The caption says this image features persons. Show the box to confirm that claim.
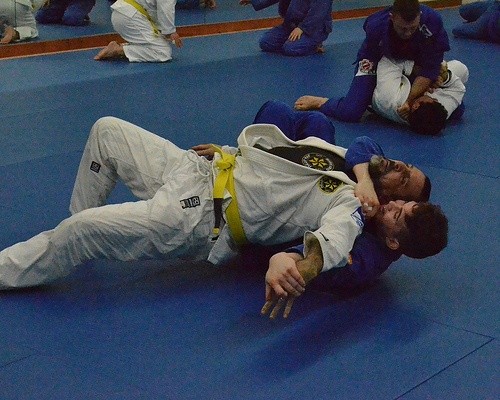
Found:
[94,0,183,62]
[294,0,451,122]
[372,54,469,136]
[452,0,500,42]
[36,0,95,26]
[253,100,449,301]
[0,116,431,318]
[240,0,333,56]
[0,0,39,44]
